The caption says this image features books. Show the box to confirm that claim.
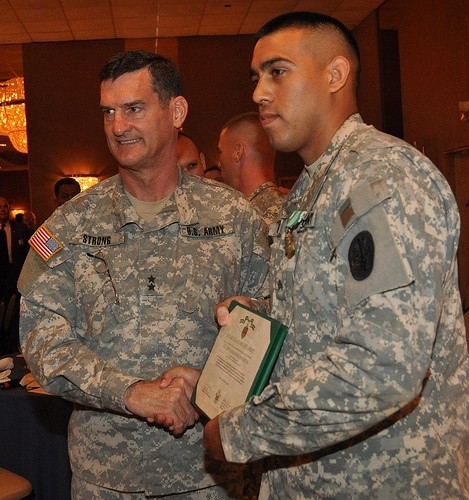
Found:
[190,299,288,424]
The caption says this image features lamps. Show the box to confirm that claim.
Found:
[65,176,98,193]
[0,77,28,153]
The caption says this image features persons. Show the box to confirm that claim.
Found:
[54,178,80,207]
[0,197,36,356]
[203,11,469,500]
[17,49,272,500]
[217,112,288,224]
[177,132,205,178]
